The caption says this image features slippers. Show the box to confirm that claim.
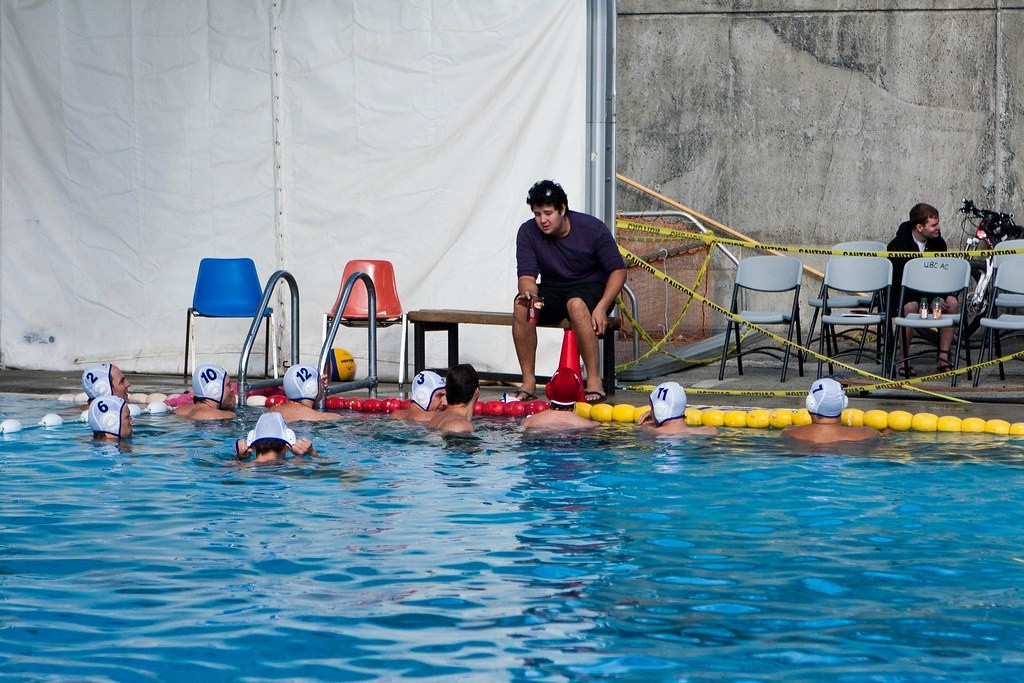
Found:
[513,390,539,400]
[898,366,917,376]
[585,390,607,403]
[937,365,952,373]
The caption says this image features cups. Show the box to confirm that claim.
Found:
[528,296,543,324]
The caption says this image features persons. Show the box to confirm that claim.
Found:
[174,364,237,419]
[389,371,448,421]
[887,203,959,376]
[81,363,130,402]
[269,363,342,423]
[429,363,480,432]
[88,395,134,439]
[235,411,316,461]
[780,377,882,443]
[521,367,600,428]
[508,180,625,402]
[637,382,719,436]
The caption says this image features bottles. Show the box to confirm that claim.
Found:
[920,298,929,319]
[933,297,941,319]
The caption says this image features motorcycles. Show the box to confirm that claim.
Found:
[914,199,1024,345]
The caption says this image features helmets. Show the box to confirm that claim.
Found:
[330,348,355,383]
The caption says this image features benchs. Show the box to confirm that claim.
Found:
[408,307,622,397]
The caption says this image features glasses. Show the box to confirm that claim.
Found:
[528,189,552,199]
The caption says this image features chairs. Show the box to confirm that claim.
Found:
[322,258,408,386]
[185,257,279,376]
[817,256,895,380]
[889,258,976,387]
[973,260,1024,388]
[718,255,805,382]
[988,238,1024,360]
[802,242,887,364]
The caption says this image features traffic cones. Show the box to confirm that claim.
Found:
[559,329,585,403]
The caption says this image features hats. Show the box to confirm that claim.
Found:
[283,364,319,401]
[89,395,126,435]
[82,363,113,401]
[650,382,687,425]
[545,367,583,405]
[411,371,446,411]
[192,364,227,403]
[806,378,849,416]
[246,412,296,449]
